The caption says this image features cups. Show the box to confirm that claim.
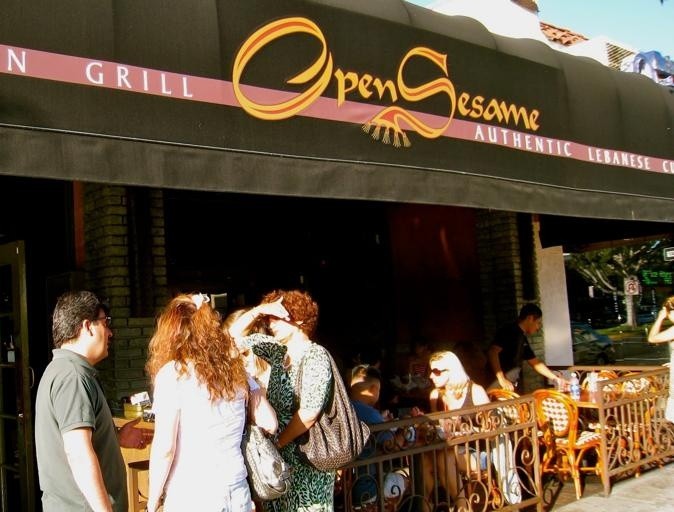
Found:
[130,390,152,422]
[587,372,598,391]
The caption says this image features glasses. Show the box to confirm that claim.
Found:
[88,315,112,327]
[427,368,446,376]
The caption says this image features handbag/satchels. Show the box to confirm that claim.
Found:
[292,344,371,472]
[241,424,292,502]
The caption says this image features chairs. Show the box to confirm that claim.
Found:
[486,370,659,501]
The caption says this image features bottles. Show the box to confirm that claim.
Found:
[554,373,565,393]
[569,373,581,402]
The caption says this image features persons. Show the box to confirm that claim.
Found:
[641,290,674,432]
[220,305,275,392]
[145,291,258,512]
[230,287,342,512]
[487,302,573,397]
[29,287,133,512]
[345,363,413,506]
[411,349,516,512]
